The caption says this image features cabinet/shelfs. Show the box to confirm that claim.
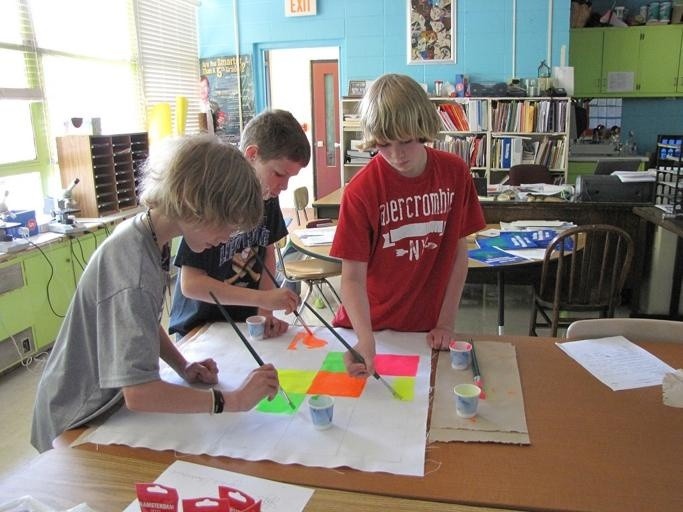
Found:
[339,96,570,185]
[56,132,150,218]
[568,155,650,185]
[568,24,683,98]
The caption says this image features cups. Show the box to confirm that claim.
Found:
[307,395,335,432]
[453,382,482,419]
[434,80,443,97]
[448,341,473,371]
[245,316,267,342]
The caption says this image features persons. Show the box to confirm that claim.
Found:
[328,72,487,379]
[168,108,311,342]
[29,129,280,453]
[200,75,220,133]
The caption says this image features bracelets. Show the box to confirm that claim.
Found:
[210,387,225,415]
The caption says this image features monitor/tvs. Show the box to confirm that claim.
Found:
[593,158,641,175]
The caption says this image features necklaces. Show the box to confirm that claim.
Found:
[146,207,171,317]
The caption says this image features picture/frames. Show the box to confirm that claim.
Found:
[406,0,457,65]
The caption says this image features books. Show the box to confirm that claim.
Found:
[343,100,567,170]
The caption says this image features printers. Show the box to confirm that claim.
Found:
[576,175,657,202]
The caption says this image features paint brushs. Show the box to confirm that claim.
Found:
[304,301,400,399]
[470,338,486,400]
[254,250,314,336]
[210,292,295,410]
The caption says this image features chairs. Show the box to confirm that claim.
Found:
[566,318,683,341]
[275,235,342,326]
[294,186,309,225]
[529,225,635,338]
[509,164,550,185]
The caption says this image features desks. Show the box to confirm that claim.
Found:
[0,449,519,511]
[312,187,652,284]
[632,207,683,316]
[284,222,586,328]
[50,321,683,511]
